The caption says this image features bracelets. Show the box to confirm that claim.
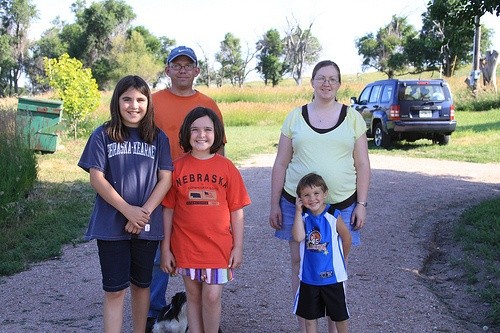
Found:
[356,201,368,207]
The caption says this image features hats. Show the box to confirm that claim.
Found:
[166,45,197,65]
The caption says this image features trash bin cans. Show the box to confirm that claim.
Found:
[16,96,61,153]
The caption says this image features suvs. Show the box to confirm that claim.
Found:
[349,78,457,149]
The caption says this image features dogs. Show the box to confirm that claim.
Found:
[154,291,189,333]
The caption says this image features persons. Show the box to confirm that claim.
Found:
[160,106,252,333]
[290,173,352,333]
[270,60,370,333]
[78,75,175,333]
[145,46,227,333]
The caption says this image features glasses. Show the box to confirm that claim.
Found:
[315,76,338,85]
[169,64,197,72]
